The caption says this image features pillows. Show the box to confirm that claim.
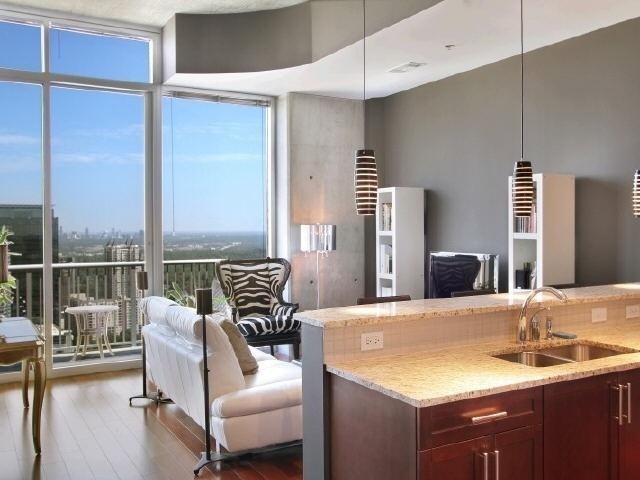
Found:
[214,315,259,376]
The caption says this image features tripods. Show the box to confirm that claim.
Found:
[128,291,161,405]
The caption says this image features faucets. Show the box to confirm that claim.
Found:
[514,286,569,344]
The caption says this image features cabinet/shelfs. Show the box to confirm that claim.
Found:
[375,185,425,300]
[507,173,576,292]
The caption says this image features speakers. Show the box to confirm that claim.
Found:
[137,271,149,289]
[196,289,213,314]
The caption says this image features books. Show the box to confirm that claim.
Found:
[514,206,536,290]
[380,202,396,296]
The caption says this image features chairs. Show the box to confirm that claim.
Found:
[429,252,481,298]
[216,258,301,360]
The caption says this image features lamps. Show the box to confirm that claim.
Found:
[631,170,640,219]
[300,223,337,311]
[129,272,159,408]
[511,1,533,217]
[353,0,378,216]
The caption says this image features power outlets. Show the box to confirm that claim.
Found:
[360,331,384,351]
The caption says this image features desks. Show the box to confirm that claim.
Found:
[1,316,46,453]
[64,304,120,362]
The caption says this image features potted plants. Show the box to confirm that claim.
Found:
[0,224,17,308]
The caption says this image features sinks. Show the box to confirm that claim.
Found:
[487,341,573,368]
[534,334,640,364]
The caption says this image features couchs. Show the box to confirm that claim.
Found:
[138,296,302,456]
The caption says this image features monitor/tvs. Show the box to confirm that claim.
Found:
[427,251,499,300]
